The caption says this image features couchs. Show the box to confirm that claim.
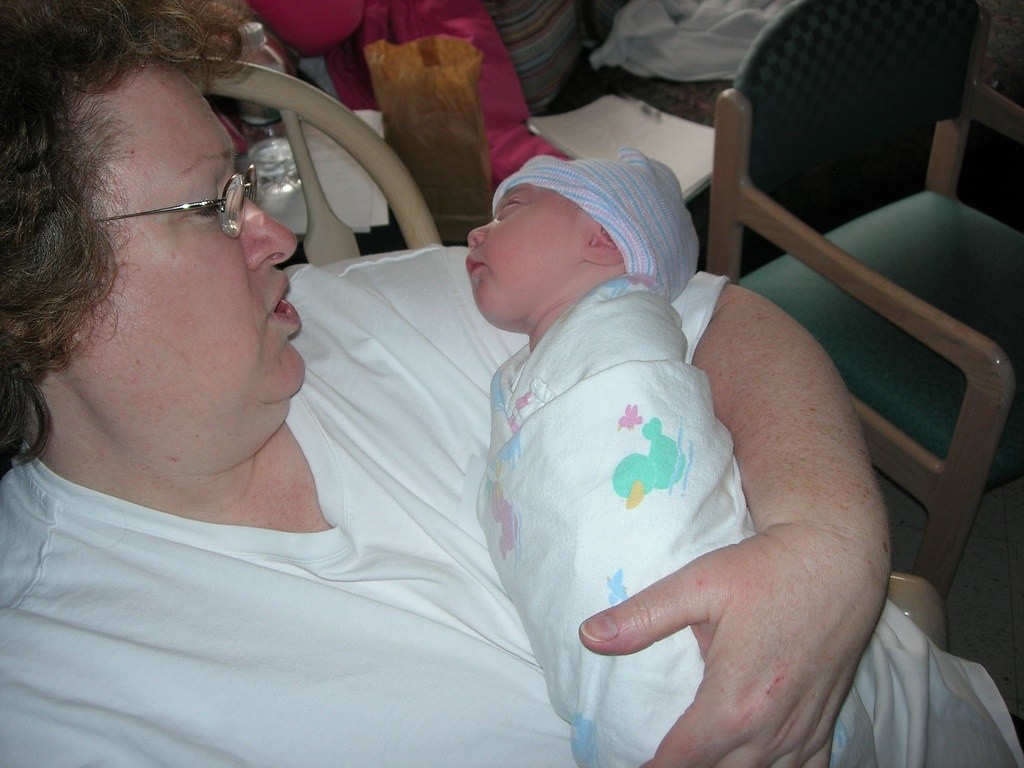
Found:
[481,0,1024,274]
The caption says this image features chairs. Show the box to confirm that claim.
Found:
[706,0,1024,598]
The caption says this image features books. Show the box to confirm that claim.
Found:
[528,97,716,210]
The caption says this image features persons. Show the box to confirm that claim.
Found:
[0,0,1023,768]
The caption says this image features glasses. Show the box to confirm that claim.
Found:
[91,162,267,239]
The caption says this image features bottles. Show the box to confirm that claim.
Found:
[234,21,303,196]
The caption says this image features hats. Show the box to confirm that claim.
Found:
[492,145,700,297]
